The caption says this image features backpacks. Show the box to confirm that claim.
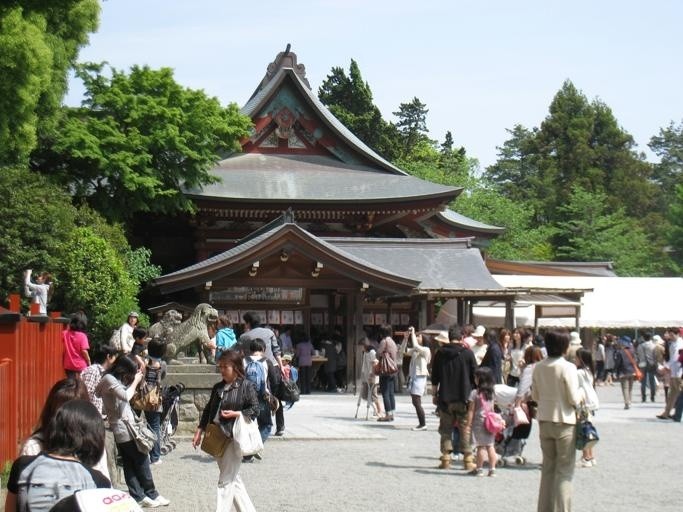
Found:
[245,356,268,392]
[288,365,298,381]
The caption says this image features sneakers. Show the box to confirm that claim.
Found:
[577,457,597,467]
[439,461,450,467]
[378,416,391,421]
[154,496,170,506]
[464,462,495,477]
[139,496,159,507]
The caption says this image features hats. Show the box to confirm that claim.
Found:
[282,354,291,360]
[50,488,143,512]
[571,331,581,344]
[471,325,485,338]
[619,336,633,348]
[434,332,451,344]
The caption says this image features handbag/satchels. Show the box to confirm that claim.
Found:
[233,411,263,457]
[376,352,398,374]
[277,377,300,402]
[636,369,643,379]
[201,424,232,457]
[485,412,504,433]
[580,369,599,411]
[132,386,161,409]
[576,421,598,450]
[123,410,159,454]
[514,407,529,428]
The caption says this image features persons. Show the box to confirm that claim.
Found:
[5,312,167,511]
[361,322,682,511]
[23,269,50,316]
[194,312,347,511]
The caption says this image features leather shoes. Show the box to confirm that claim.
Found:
[412,426,427,431]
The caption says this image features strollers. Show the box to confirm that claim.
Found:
[157,382,186,455]
[494,402,533,467]
